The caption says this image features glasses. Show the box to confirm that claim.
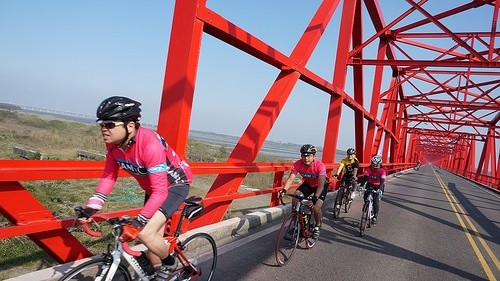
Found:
[100,121,124,129]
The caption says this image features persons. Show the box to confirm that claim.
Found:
[76,97,192,280]
[335,148,359,209]
[277,144,330,239]
[361,156,386,223]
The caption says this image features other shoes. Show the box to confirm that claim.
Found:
[336,200,339,209]
[362,205,367,213]
[371,216,377,224]
[350,190,357,199]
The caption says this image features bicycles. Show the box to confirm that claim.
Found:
[274,188,323,267]
[333,175,357,219]
[58,195,217,281]
[358,188,383,238]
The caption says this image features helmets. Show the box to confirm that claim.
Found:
[346,148,355,155]
[96,96,142,123]
[300,144,317,154]
[371,156,382,165]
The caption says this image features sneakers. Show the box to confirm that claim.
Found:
[312,224,321,239]
[153,256,183,281]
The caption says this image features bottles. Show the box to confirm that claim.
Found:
[137,251,155,276]
[302,212,308,226]
